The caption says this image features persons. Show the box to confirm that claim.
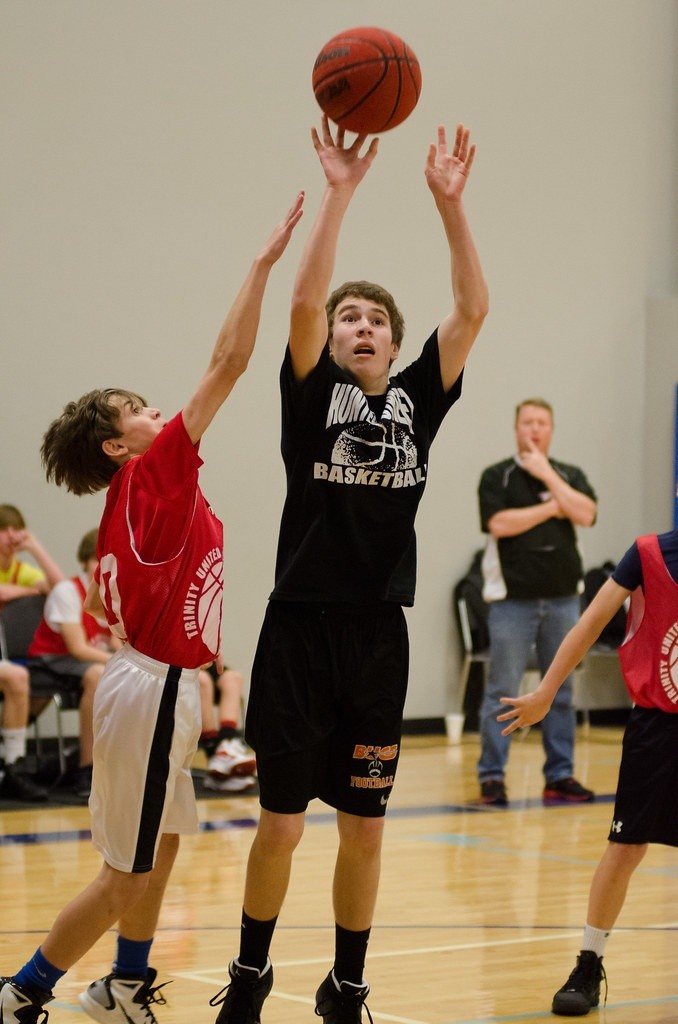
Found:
[0,505,259,801]
[210,112,488,1024]
[478,398,597,806]
[0,189,308,1024]
[497,483,678,1016]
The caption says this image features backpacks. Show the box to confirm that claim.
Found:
[579,558,627,650]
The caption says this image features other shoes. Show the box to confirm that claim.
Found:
[76,765,91,796]
[464,717,479,733]
[0,756,47,802]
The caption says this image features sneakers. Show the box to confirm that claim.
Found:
[202,739,256,792]
[0,976,56,1024]
[314,968,373,1024]
[551,950,608,1016]
[542,778,594,802]
[209,954,273,1024]
[480,781,507,804]
[79,967,175,1024]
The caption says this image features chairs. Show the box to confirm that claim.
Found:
[0,592,80,789]
[454,599,587,735]
